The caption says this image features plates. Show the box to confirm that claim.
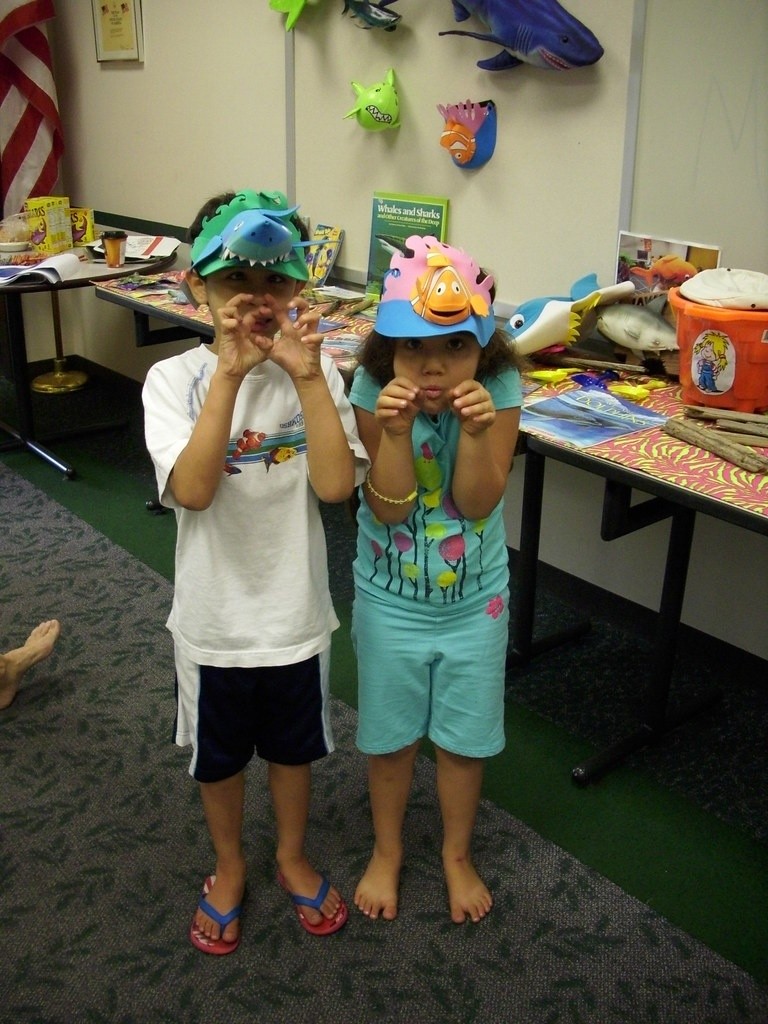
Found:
[0,242,29,251]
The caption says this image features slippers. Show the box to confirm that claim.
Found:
[191,875,238,953]
[275,865,348,934]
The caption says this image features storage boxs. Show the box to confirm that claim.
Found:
[70,208,95,246]
[668,287,768,415]
[24,196,74,255]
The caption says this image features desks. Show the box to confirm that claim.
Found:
[95,269,646,673]
[0,251,178,394]
[526,373,768,788]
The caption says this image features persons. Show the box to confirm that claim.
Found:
[341,233,523,924]
[0,619,59,709]
[139,187,373,956]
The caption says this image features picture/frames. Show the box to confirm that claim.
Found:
[91,0,144,63]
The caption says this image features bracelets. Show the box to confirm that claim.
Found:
[363,467,422,505]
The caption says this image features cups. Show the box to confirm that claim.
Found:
[100,229,128,268]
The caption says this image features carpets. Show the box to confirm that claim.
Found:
[0,461,768,1024]
